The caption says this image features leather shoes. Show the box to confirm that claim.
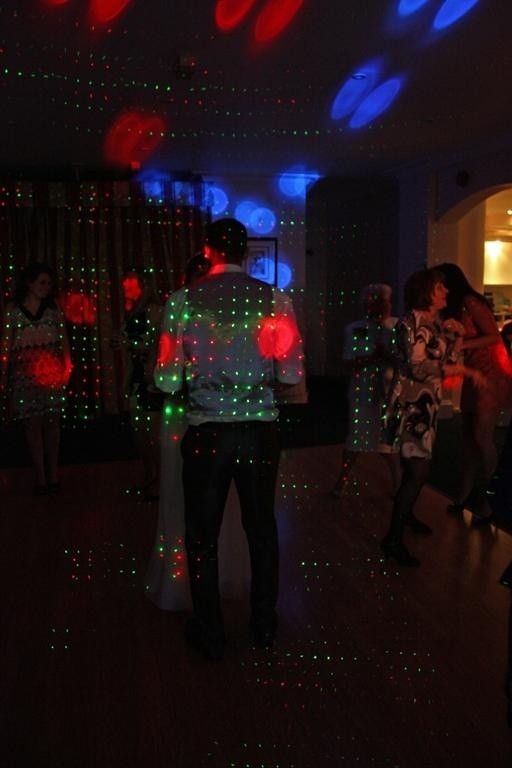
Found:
[48,483,61,494]
[30,464,47,496]
[183,619,222,661]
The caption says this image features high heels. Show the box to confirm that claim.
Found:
[446,495,478,514]
[380,537,422,567]
[403,509,432,536]
[472,509,499,529]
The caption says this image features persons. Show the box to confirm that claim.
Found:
[106,253,164,499]
[1,264,73,490]
[329,284,400,501]
[141,251,252,614]
[375,270,490,570]
[431,260,511,528]
[157,215,308,658]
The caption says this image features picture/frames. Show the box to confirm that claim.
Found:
[240,237,277,285]
